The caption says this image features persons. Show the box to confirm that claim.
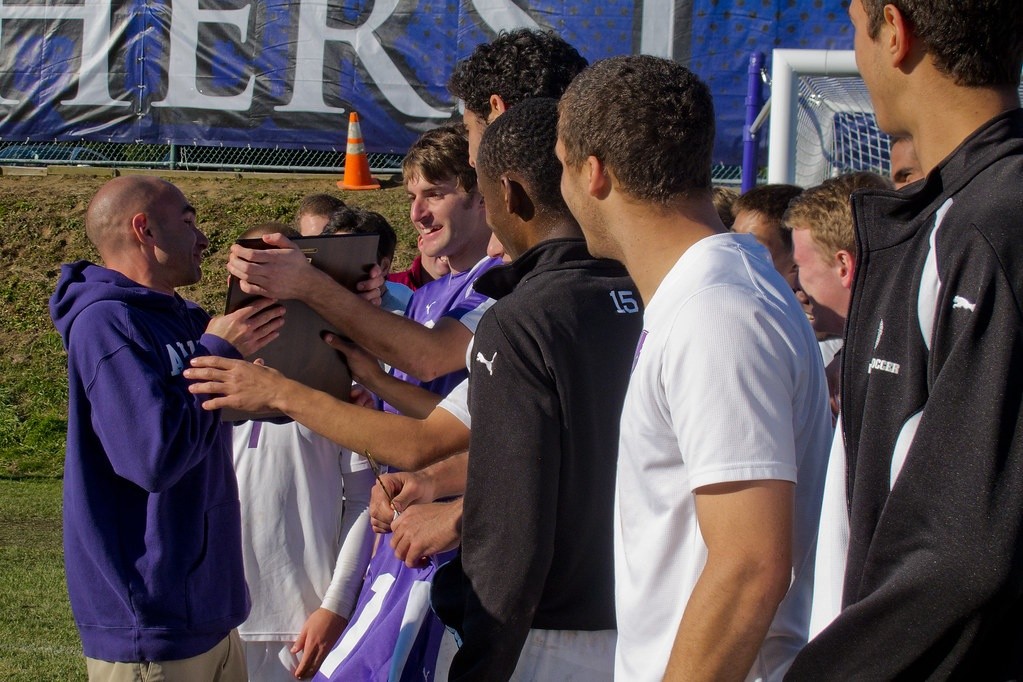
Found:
[48,174,287,682]
[783,0,1023,682]
[181,28,922,682]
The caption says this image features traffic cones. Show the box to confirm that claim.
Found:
[337,111,381,190]
